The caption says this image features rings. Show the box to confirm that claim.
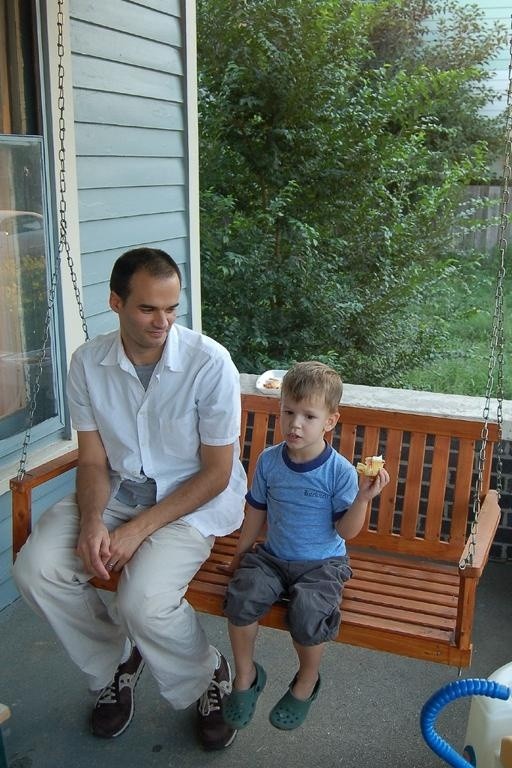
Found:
[107,562,115,569]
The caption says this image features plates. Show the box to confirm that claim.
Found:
[256,369,288,394]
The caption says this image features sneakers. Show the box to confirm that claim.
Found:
[88,636,146,739]
[196,645,239,752]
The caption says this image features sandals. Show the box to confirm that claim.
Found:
[269,667,323,732]
[220,659,269,730]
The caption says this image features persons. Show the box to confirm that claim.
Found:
[219,361,389,730]
[12,246,249,750]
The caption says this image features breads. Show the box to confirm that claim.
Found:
[358,456,385,477]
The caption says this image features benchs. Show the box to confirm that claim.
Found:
[9,390,503,670]
